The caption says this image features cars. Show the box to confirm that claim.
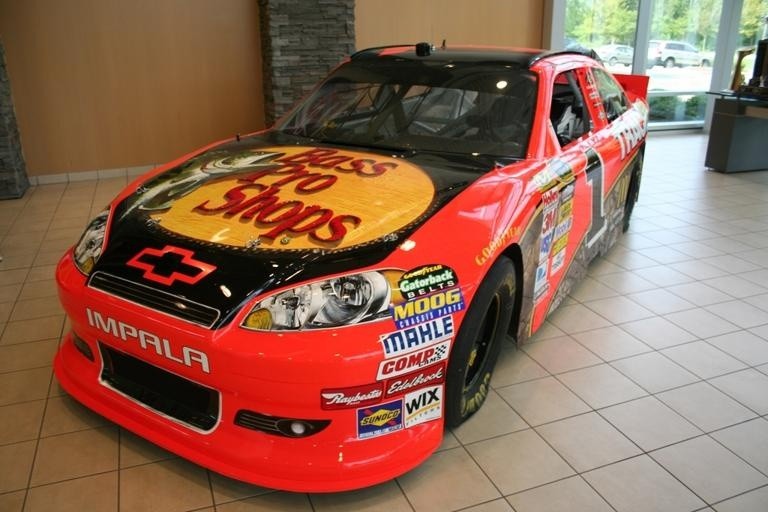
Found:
[591,42,635,67]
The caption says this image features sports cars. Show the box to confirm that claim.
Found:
[46,38,657,500]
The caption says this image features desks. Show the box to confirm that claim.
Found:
[704,90,768,174]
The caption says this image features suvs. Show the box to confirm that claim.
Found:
[645,38,711,69]
